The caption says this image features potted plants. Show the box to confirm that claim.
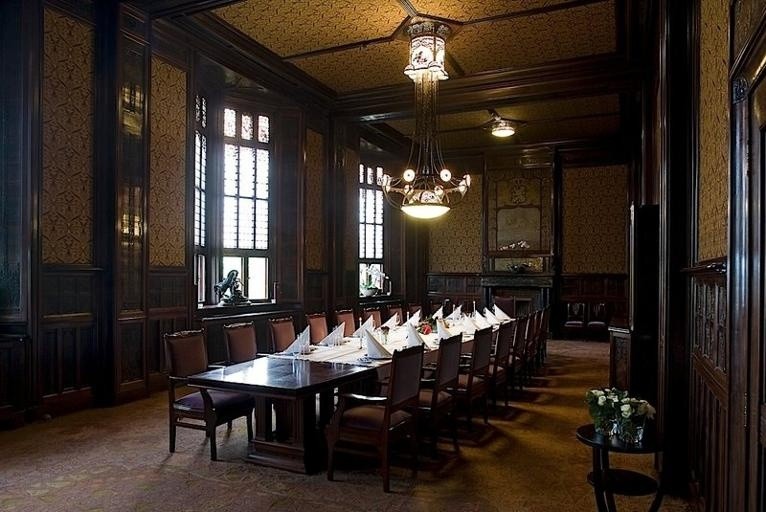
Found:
[358,280,378,297]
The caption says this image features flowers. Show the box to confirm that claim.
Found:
[584,388,656,444]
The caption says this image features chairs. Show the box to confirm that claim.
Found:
[322,304,549,492]
[164,303,448,459]
[563,301,606,341]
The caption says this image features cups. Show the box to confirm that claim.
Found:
[293,306,495,360]
[292,359,310,382]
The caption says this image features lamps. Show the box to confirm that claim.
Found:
[382,14,471,220]
[483,109,526,137]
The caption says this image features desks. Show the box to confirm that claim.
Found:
[577,424,670,512]
[188,316,516,475]
